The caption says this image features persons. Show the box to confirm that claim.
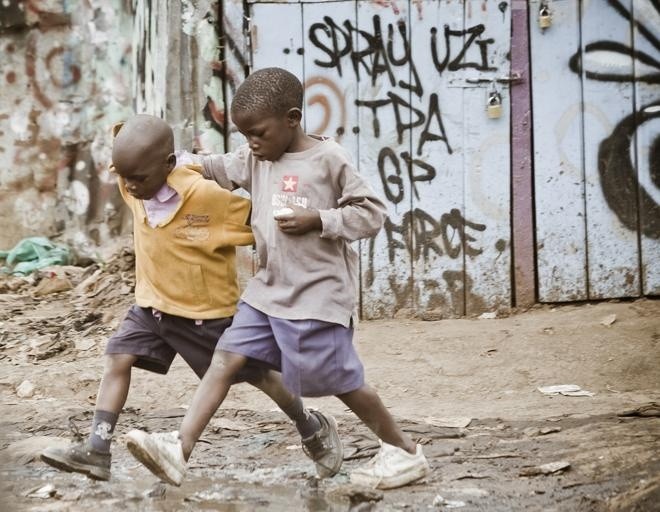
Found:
[32,110,345,484]
[122,65,432,493]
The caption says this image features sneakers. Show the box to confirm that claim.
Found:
[301,408,344,477]
[125,429,189,487]
[349,438,430,490]
[40,440,111,480]
